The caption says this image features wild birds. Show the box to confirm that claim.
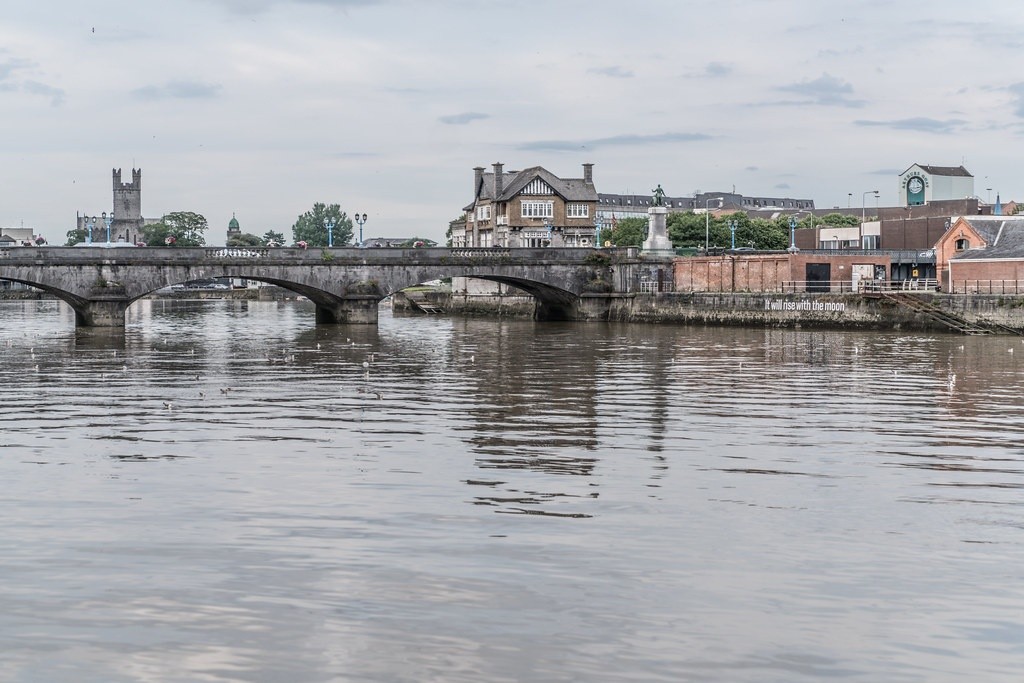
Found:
[0,311,1024,451]
[90,27,96,33]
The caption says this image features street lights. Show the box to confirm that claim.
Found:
[787,215,800,253]
[798,211,813,229]
[848,192,852,209]
[101,211,115,245]
[323,216,339,246]
[726,220,740,250]
[704,196,725,252]
[874,195,880,217]
[354,213,368,245]
[861,188,879,250]
[987,188,993,204]
[542,216,554,245]
[591,214,605,248]
[84,215,97,243]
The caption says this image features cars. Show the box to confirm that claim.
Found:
[734,246,756,251]
